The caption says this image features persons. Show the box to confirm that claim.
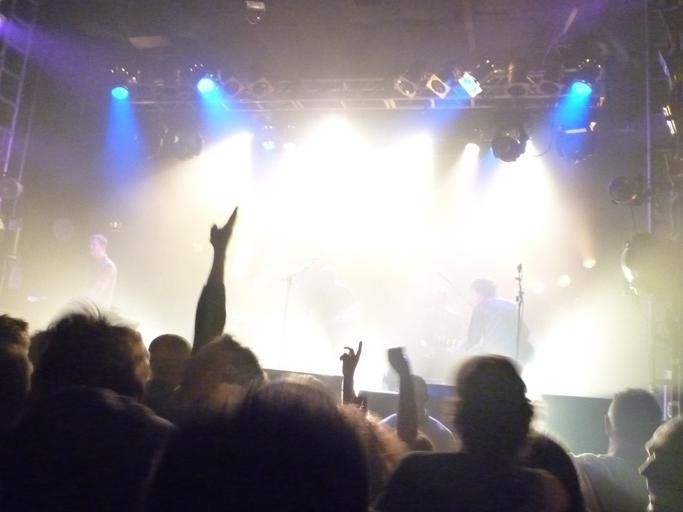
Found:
[85,233,115,317]
[185,205,236,354]
[339,341,584,512]
[571,388,682,512]
[0,315,369,512]
[466,279,534,378]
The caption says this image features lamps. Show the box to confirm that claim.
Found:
[572,62,602,94]
[110,66,129,100]
[469,59,496,87]
[184,66,213,93]
